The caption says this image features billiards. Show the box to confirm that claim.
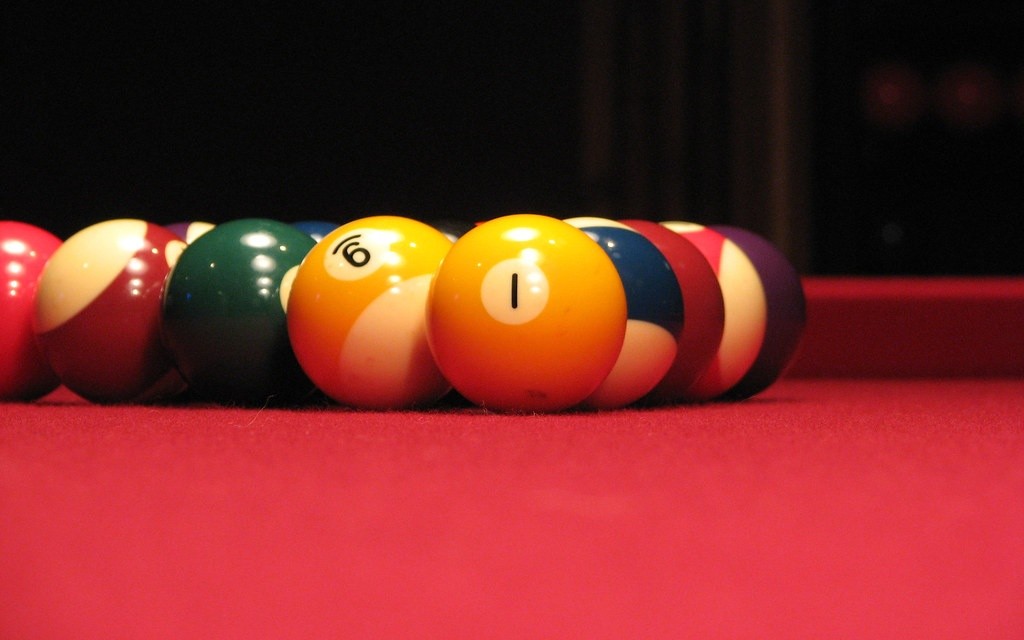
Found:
[430,214,626,410]
[563,214,813,406]
[0,212,451,412]
